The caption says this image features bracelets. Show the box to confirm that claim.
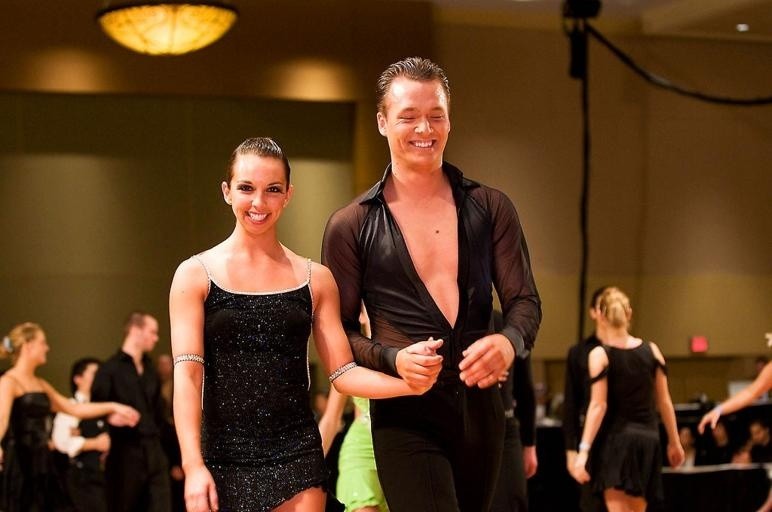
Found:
[578,443,591,451]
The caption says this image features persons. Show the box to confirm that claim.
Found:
[679,322,772,512]
[318,300,389,512]
[0,312,185,512]
[493,309,539,512]
[574,287,685,512]
[170,137,438,512]
[320,58,543,512]
[564,288,605,511]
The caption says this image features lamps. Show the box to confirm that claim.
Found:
[95,0,239,58]
